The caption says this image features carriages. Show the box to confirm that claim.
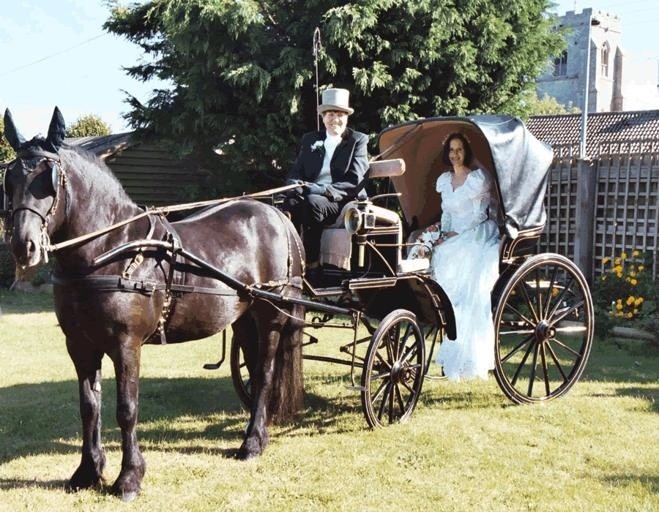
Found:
[0,108,597,509]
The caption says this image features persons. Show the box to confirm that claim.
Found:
[403,133,501,383]
[283,87,372,285]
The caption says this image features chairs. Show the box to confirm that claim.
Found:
[300,158,406,271]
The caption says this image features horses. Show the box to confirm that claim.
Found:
[2,105,309,504]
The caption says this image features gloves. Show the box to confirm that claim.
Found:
[288,178,326,195]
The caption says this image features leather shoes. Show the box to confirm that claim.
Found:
[305,264,325,283]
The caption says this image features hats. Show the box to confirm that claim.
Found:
[318,88,354,115]
